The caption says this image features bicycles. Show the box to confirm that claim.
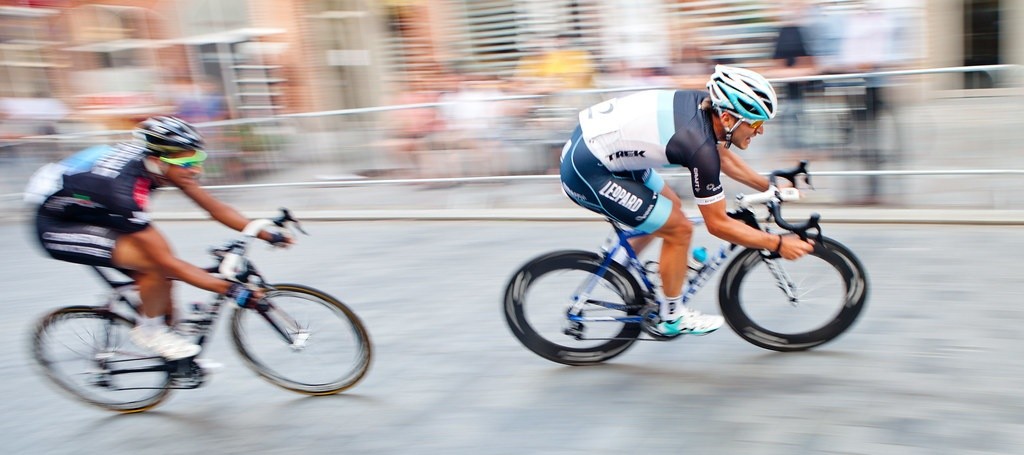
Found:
[30,200,371,414]
[502,158,868,366]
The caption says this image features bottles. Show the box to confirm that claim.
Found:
[681,246,708,292]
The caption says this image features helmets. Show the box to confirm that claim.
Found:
[134,117,205,154]
[706,63,779,121]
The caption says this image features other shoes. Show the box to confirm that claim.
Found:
[132,323,201,361]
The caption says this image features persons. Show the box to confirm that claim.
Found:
[1,48,251,186]
[27,117,293,379]
[381,0,894,199]
[559,65,814,335]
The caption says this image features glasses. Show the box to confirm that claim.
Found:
[154,149,209,166]
[745,120,765,131]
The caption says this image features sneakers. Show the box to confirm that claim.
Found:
[655,305,725,338]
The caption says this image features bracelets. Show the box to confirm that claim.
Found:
[775,234,783,253]
